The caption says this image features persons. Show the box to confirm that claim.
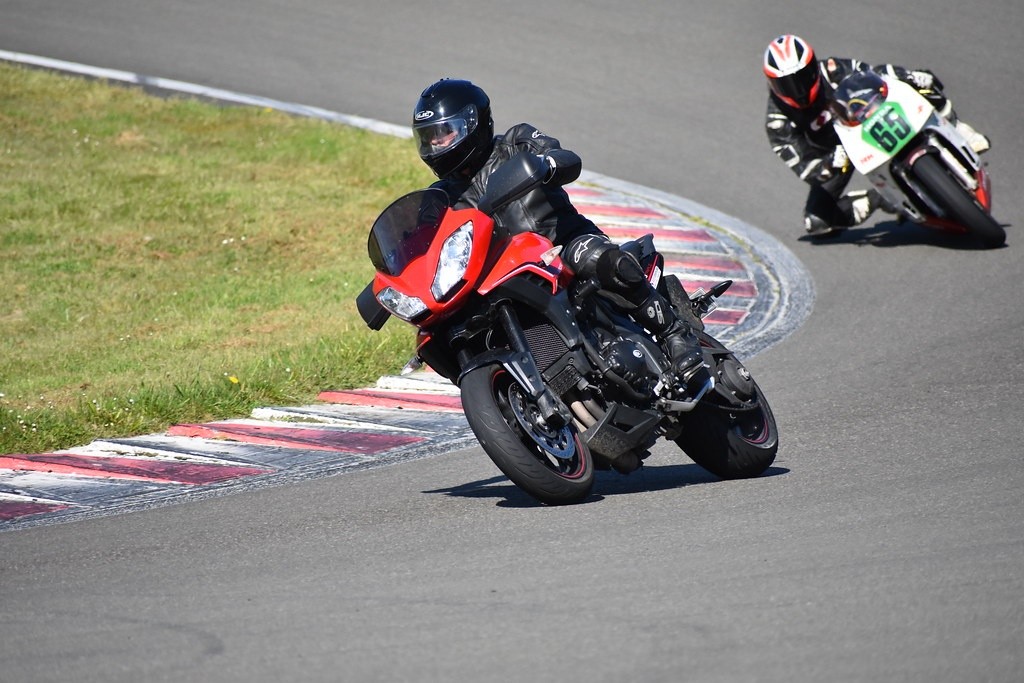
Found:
[411,77,707,386]
[763,34,934,235]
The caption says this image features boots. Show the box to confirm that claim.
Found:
[627,290,703,376]
[956,120,990,154]
[848,189,897,230]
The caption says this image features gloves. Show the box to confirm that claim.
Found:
[832,144,849,168]
[906,70,934,89]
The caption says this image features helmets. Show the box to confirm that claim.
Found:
[412,78,494,179]
[762,34,822,109]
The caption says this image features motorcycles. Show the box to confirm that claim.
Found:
[829,71,1007,248]
[356,152,778,503]
